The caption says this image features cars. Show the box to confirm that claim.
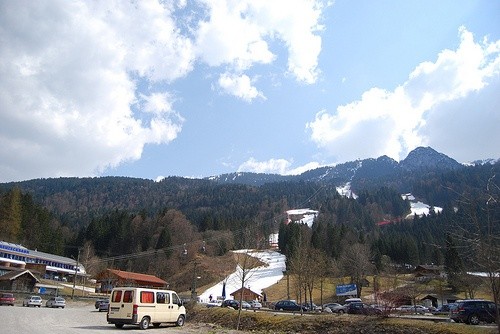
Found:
[0,293,16,307]
[99,301,109,312]
[220,299,240,310]
[320,297,455,315]
[268,301,278,310]
[302,302,317,308]
[22,295,43,308]
[238,300,251,310]
[95,299,106,309]
[247,300,262,310]
[45,297,66,309]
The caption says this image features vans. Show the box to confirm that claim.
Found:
[205,299,226,308]
[106,286,187,330]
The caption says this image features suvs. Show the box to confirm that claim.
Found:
[449,299,500,326]
[273,300,308,314]
[172,296,191,305]
